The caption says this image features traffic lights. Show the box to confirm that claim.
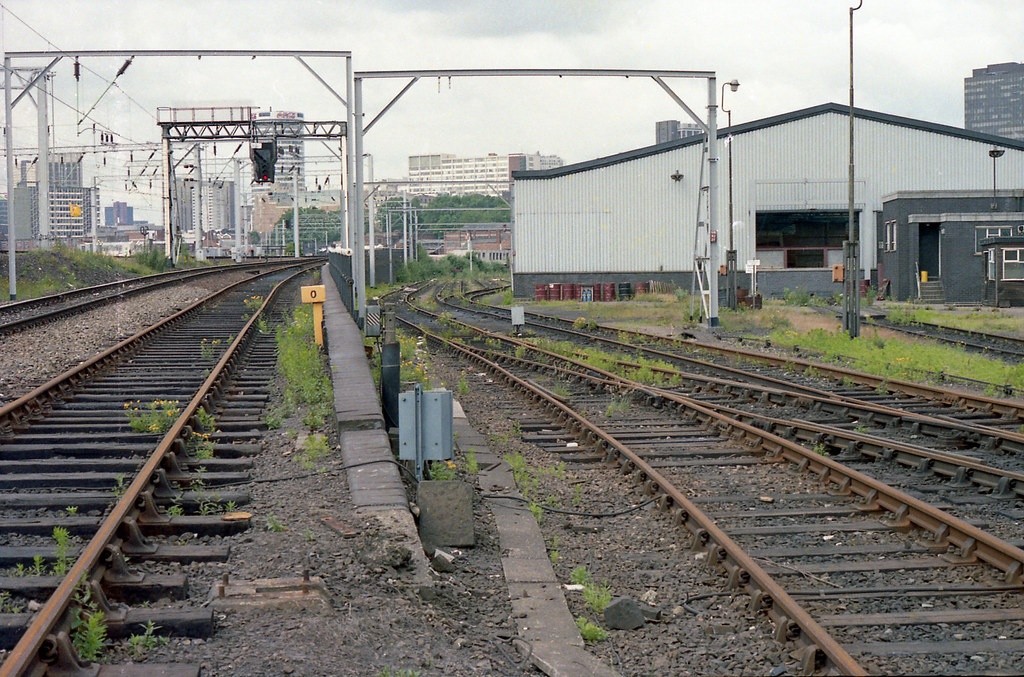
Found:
[259,162,270,181]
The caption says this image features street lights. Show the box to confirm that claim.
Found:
[721,78,738,308]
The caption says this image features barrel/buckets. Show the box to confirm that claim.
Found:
[635,282,649,294]
[846,279,870,298]
[535,284,548,301]
[594,284,600,302]
[562,283,573,300]
[574,284,581,299]
[746,294,763,308]
[603,282,615,301]
[619,282,630,301]
[549,283,561,301]
